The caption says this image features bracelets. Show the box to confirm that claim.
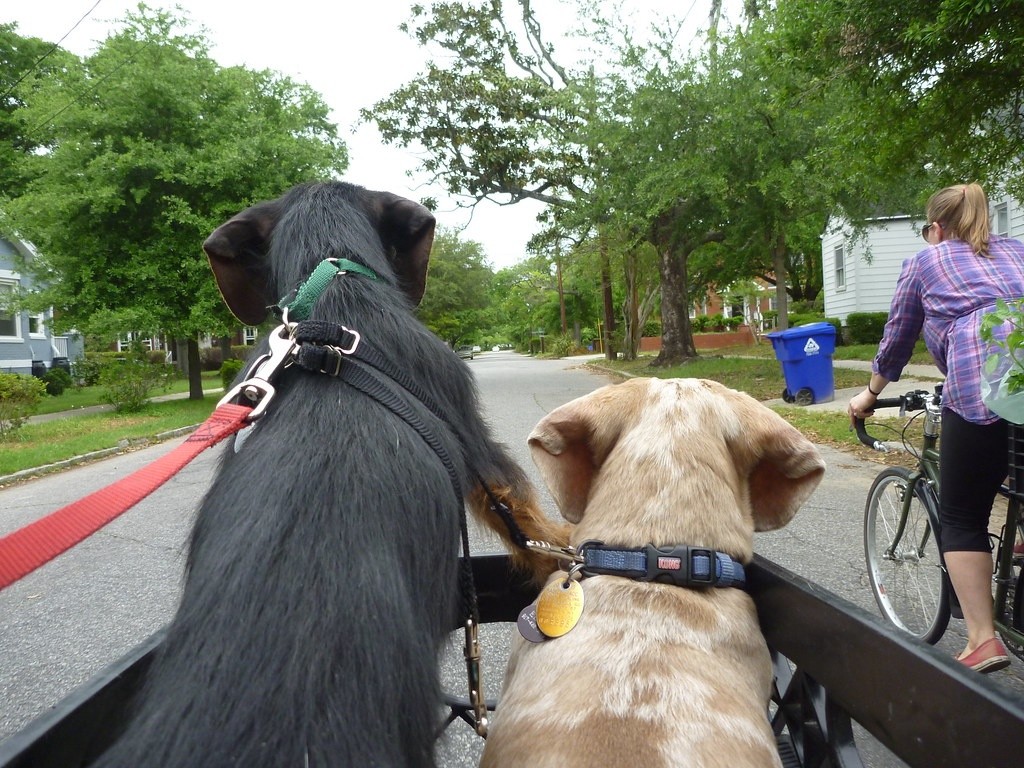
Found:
[868,383,880,395]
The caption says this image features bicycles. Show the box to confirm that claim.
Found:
[851,384,1024,670]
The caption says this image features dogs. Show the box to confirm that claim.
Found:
[477,373,828,768]
[86,178,574,768]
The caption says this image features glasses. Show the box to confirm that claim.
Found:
[922,223,945,241]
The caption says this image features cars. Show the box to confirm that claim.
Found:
[472,346,481,353]
[492,346,501,352]
[456,345,474,360]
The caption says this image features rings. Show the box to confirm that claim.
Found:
[854,412,857,417]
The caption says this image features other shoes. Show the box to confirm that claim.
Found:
[1014,539,1024,557]
[955,638,1011,674]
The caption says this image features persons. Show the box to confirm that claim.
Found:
[848,182,1024,672]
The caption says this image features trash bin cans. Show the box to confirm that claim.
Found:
[589,345,593,350]
[53,357,71,375]
[31,360,47,378]
[765,323,839,404]
